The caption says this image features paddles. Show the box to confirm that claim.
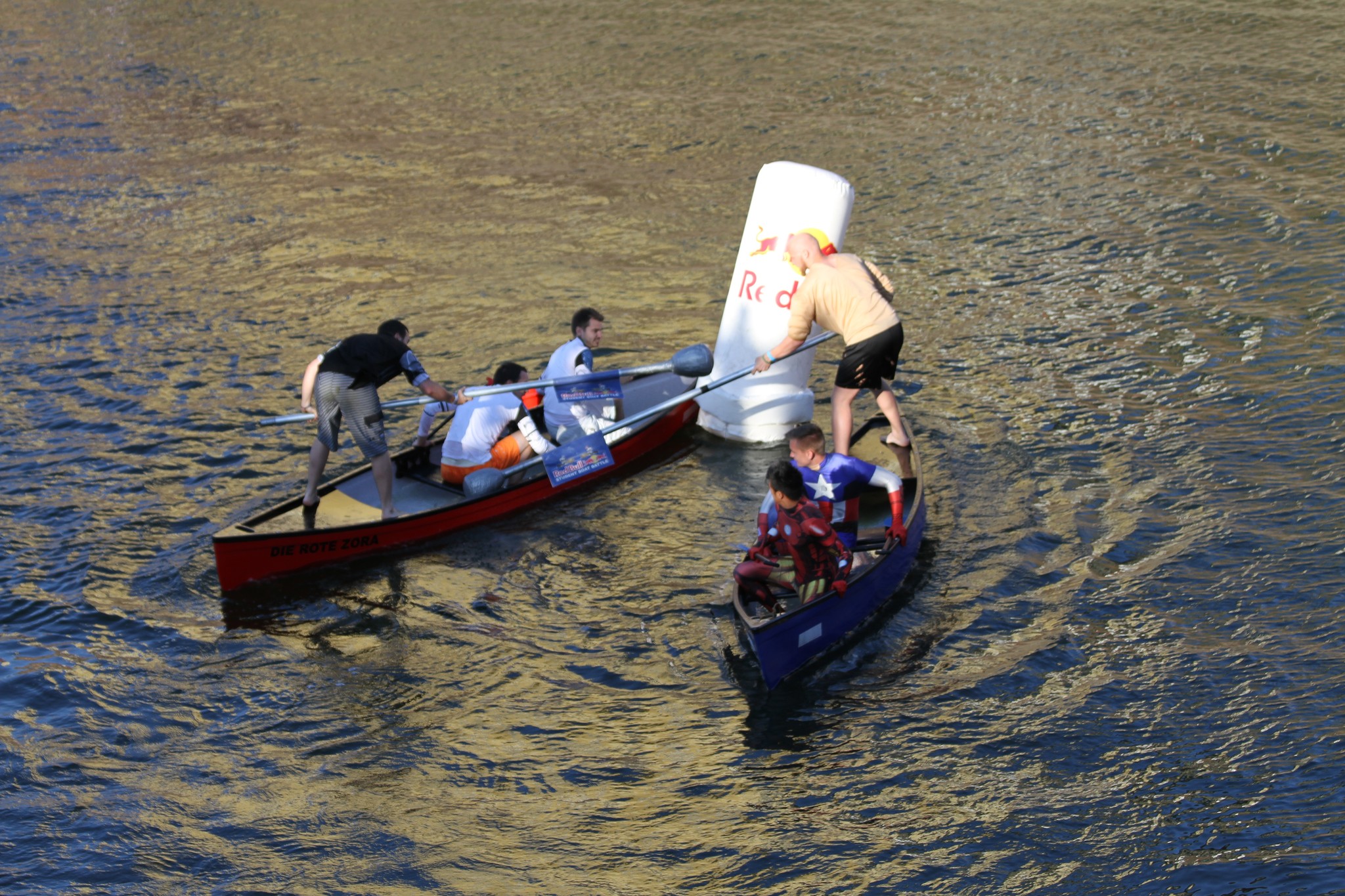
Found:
[874,515,907,554]
[413,411,456,447]
[726,542,780,568]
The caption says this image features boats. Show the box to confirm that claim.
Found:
[732,412,926,687]
[210,363,705,594]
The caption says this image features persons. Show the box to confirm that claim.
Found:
[411,363,557,487]
[752,233,910,456]
[757,423,907,550]
[535,306,633,446]
[300,319,473,517]
[732,460,854,619]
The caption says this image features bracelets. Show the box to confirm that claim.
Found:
[767,350,776,362]
[302,404,312,410]
[452,393,458,405]
[761,355,772,365]
